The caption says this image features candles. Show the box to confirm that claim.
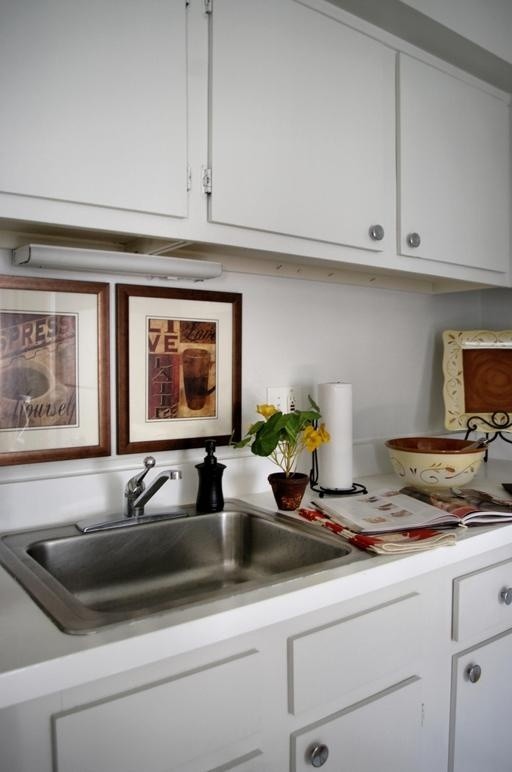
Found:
[318,382,355,493]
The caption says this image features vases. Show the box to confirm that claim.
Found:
[265,469,308,511]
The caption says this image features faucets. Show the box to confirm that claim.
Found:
[121,456,183,522]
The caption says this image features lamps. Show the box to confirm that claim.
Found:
[8,239,226,286]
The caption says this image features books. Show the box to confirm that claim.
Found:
[308,483,512,536]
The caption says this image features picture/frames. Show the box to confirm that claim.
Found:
[0,271,114,465]
[116,281,242,454]
[442,327,511,429]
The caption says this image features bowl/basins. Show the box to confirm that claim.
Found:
[384,437,488,497]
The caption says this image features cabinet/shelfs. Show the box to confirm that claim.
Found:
[277,559,443,772]
[2,0,198,260]
[2,607,277,772]
[154,1,512,296]
[445,543,510,772]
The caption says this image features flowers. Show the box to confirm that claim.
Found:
[230,397,331,475]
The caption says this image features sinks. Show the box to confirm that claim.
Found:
[15,503,359,621]
